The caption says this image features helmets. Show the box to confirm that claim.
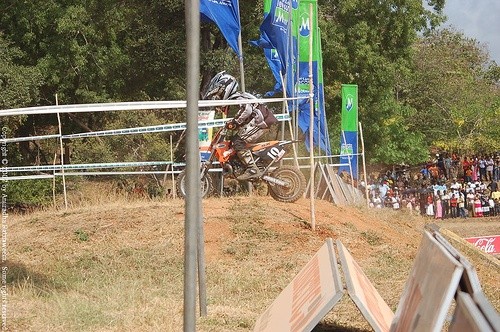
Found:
[204,71,238,100]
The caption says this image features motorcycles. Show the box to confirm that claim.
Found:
[176,123,306,205]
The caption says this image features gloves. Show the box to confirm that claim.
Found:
[226,118,240,130]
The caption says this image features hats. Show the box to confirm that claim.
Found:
[453,178,456,180]
[467,182,471,185]
[475,182,479,184]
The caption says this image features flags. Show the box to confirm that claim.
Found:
[200,0,358,179]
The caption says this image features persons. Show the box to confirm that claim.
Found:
[339,152,500,218]
[203,70,278,180]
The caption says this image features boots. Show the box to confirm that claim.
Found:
[236,149,260,180]
